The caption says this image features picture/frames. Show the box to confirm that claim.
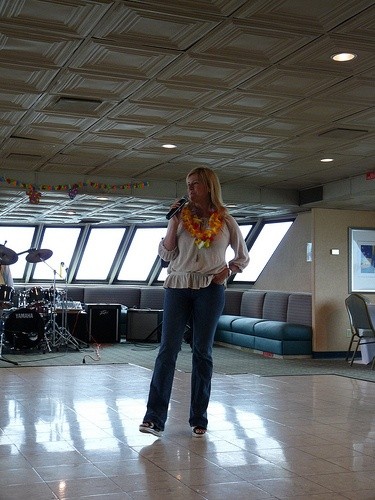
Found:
[348,226,375,294]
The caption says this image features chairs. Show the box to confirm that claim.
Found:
[345,293,375,369]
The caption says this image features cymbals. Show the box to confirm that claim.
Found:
[25,249,53,262]
[0,243,18,265]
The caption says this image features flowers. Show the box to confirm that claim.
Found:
[181,203,224,250]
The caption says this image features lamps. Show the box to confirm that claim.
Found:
[330,51,358,64]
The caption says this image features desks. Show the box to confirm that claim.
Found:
[359,304,375,365]
[56,311,86,335]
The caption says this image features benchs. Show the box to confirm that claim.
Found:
[14,285,312,360]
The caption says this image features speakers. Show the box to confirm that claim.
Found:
[85,303,121,344]
[126,308,164,343]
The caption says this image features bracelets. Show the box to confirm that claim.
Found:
[227,269,234,277]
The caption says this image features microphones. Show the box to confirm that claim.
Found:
[0,260,88,365]
[166,195,188,220]
[65,268,68,273]
[61,262,64,267]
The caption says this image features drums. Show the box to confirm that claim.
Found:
[18,291,29,306]
[0,308,45,349]
[0,284,16,304]
[44,289,58,305]
[29,286,45,306]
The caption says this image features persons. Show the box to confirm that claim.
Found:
[139,167,249,437]
[0,265,14,316]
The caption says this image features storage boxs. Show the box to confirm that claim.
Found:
[84,303,121,343]
[126,309,164,343]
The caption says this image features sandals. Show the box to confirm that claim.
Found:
[191,425,207,437]
[139,422,164,437]
